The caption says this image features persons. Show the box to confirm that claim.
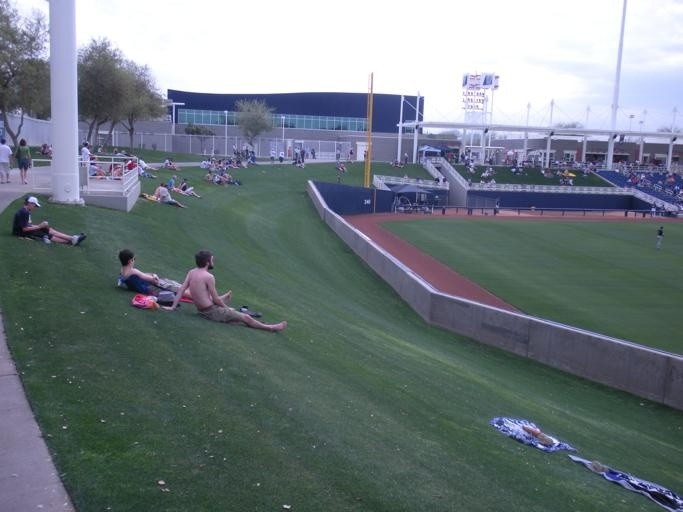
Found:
[81,141,256,208]
[336,144,367,184]
[655,225,665,251]
[446,148,682,215]
[40,144,53,159]
[0,138,13,183]
[390,152,410,169]
[14,139,32,184]
[162,251,288,332]
[13,196,87,246]
[118,249,232,304]
[269,142,319,169]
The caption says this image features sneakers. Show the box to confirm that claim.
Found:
[71,233,86,246]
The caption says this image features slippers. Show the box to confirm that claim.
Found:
[248,311,262,317]
[240,306,249,312]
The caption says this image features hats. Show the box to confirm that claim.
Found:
[25,196,40,207]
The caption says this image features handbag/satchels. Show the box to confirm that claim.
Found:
[15,150,20,159]
[159,291,176,303]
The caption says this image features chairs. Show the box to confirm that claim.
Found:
[597,171,683,205]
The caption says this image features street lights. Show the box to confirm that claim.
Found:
[280,116,285,155]
[480,127,488,165]
[607,133,617,171]
[409,123,418,162]
[221,110,228,157]
[545,130,554,168]
[668,134,676,170]
